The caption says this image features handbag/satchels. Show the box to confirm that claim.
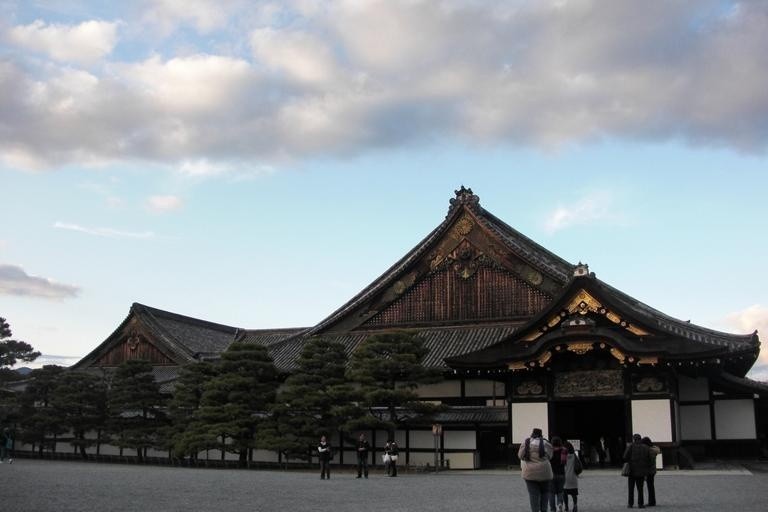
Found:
[622,463,630,477]
[383,454,398,463]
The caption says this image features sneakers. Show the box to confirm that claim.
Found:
[552,504,580,512]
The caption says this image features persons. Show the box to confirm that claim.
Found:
[517,427,554,512]
[623,433,649,508]
[562,441,584,512]
[549,437,568,511]
[597,436,608,463]
[317,434,332,480]
[354,431,371,479]
[384,438,392,476]
[0,425,14,464]
[642,435,661,506]
[391,438,399,476]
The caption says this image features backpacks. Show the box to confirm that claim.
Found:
[574,455,582,476]
